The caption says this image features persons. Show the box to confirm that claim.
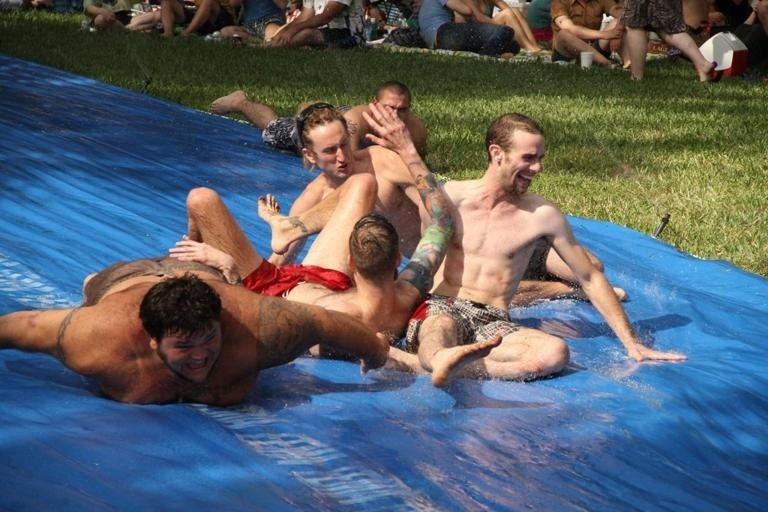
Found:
[0,258,390,407]
[385,112,689,389]
[81,0,768,87]
[208,79,428,159]
[168,102,456,360]
[266,102,628,307]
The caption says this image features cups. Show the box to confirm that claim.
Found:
[580,52,594,71]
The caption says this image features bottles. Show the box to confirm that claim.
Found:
[367,18,379,41]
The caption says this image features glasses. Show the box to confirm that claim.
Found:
[298,102,335,148]
